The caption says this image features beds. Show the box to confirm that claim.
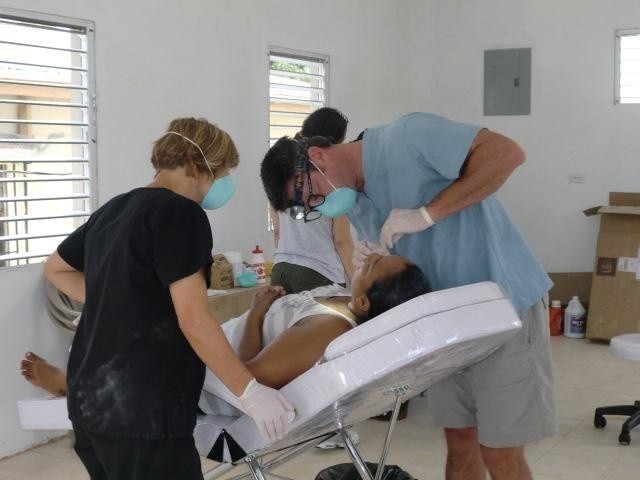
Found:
[16,282,522,479]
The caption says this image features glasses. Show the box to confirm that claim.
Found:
[303,156,326,224]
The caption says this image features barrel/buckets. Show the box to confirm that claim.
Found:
[564,295,586,338]
[550,300,562,336]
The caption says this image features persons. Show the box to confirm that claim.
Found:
[18,253,431,419]
[260,112,559,479]
[41,117,295,480]
[268,107,361,451]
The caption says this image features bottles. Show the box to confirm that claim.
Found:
[252,245,266,284]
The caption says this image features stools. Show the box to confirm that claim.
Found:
[594,333,640,445]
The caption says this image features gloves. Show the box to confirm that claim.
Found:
[352,240,380,268]
[380,205,436,254]
[238,378,295,443]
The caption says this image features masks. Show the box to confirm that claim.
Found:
[290,137,358,220]
[202,170,237,211]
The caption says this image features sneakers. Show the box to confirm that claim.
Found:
[315,430,359,448]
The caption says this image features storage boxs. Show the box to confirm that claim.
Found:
[578,191,640,341]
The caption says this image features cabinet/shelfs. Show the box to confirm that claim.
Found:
[208,287,258,326]
